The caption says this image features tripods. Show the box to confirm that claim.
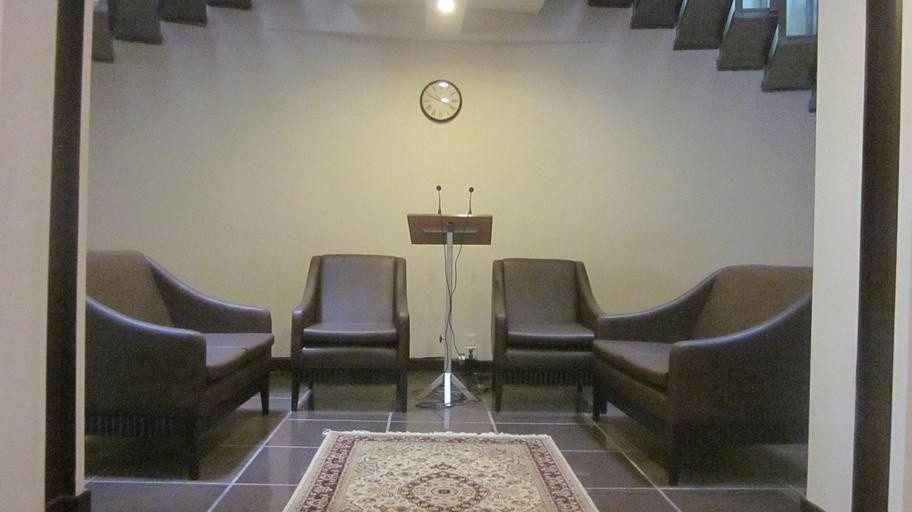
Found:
[417,232,480,406]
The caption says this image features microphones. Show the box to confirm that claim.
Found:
[468,187,474,213]
[436,186,441,214]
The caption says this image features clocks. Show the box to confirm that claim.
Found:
[419,78,464,123]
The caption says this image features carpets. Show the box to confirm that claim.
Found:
[281,426,599,511]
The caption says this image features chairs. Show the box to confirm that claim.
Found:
[81,246,274,484]
[589,261,813,490]
[287,252,414,414]
[488,256,615,425]
